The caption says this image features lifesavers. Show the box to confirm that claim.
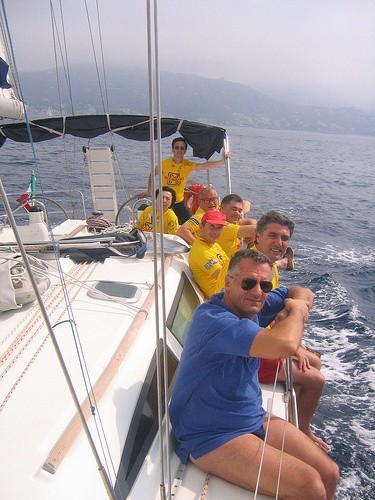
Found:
[183,184,207,214]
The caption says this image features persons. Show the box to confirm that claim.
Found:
[189,210,231,296]
[251,211,331,453]
[177,188,295,271]
[168,250,339,500]
[132,186,179,236]
[137,137,230,225]
[217,193,258,258]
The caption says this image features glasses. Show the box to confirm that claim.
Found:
[173,146,185,150]
[200,196,218,204]
[226,275,272,293]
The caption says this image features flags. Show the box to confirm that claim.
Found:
[16,176,33,213]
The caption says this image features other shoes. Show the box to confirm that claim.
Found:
[283,248,294,271]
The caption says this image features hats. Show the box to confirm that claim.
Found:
[201,211,226,226]
